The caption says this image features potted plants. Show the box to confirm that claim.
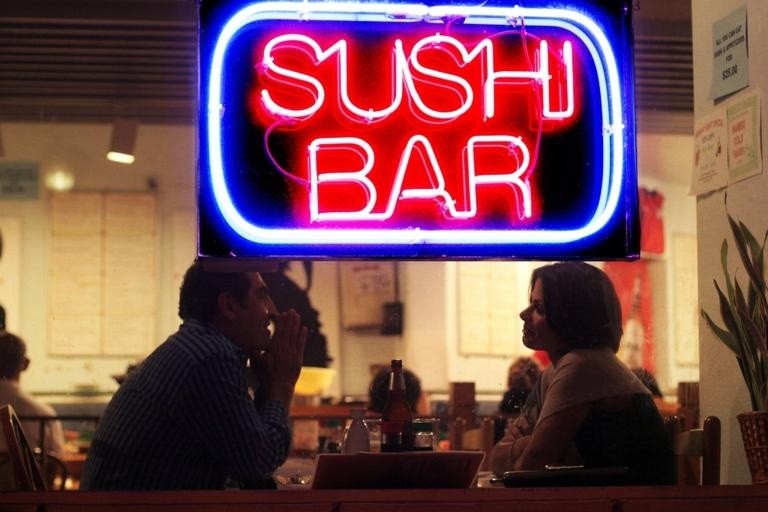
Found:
[698,179,768,484]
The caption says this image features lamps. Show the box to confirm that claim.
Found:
[104,115,140,167]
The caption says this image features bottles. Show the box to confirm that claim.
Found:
[341,408,370,453]
[381,359,414,453]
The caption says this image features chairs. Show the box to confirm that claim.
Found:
[1,403,59,491]
[665,413,723,487]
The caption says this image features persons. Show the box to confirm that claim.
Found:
[364,366,435,452]
[488,261,679,485]
[79,255,310,489]
[497,354,542,414]
[0,329,64,459]
[626,366,681,420]
